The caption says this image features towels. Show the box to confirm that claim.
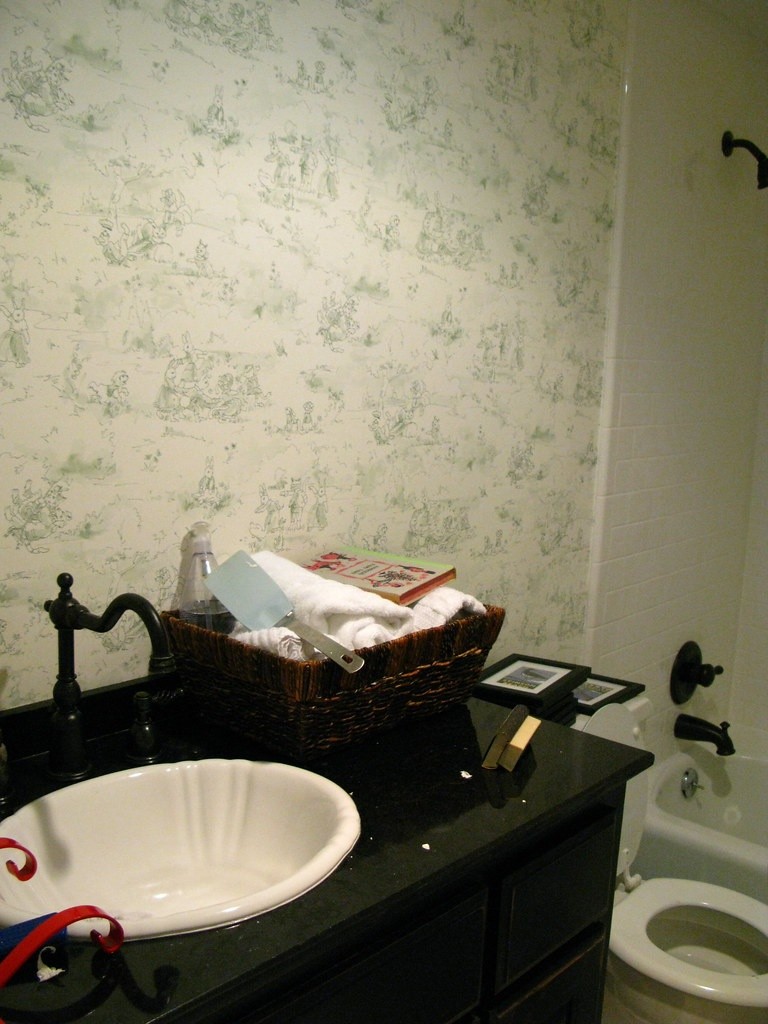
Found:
[227,542,486,661]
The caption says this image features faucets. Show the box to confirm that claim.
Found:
[39,566,176,781]
[674,713,737,757]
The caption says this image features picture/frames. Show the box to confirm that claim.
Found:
[475,653,591,704]
[574,674,648,716]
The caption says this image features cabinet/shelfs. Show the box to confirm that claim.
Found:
[193,780,631,1024]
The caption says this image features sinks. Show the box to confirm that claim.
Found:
[0,754,361,946]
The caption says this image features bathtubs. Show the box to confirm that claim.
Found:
[637,723,768,904]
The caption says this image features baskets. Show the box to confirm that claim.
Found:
[161,603,505,751]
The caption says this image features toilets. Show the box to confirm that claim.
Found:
[569,687,768,1024]
